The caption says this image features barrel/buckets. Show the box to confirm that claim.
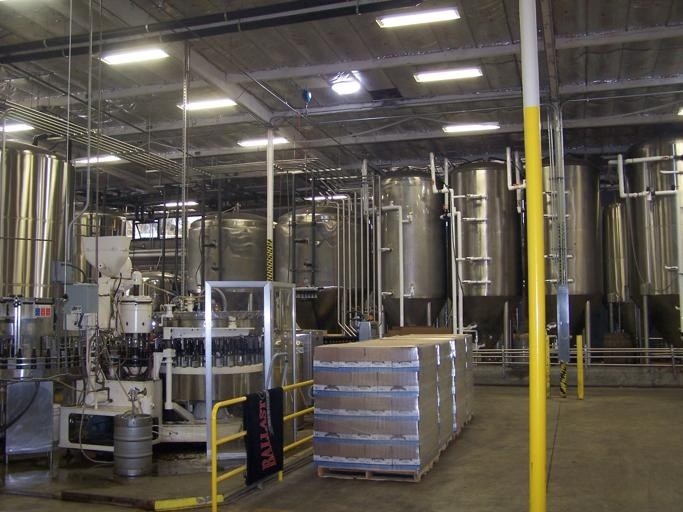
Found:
[113,414,153,476]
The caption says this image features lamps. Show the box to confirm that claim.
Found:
[237,138,290,146]
[331,81,362,94]
[100,49,170,65]
[375,6,501,133]
[176,99,237,110]
[0,123,35,133]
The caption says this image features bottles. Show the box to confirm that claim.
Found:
[1,337,253,369]
